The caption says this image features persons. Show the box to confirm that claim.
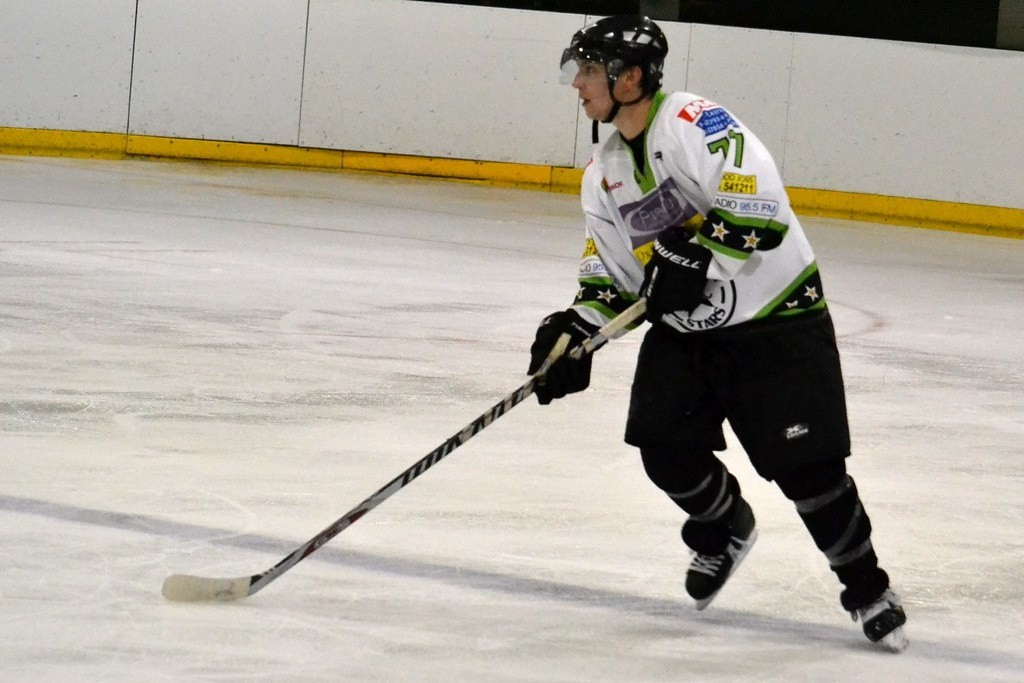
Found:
[527,13,908,652]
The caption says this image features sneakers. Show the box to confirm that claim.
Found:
[840,571,907,652]
[682,495,757,612]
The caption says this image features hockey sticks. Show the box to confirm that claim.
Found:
[160,294,650,606]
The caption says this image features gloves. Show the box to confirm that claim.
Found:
[528,309,607,404]
[638,226,713,324]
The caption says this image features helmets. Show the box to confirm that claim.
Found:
[560,14,669,87]
